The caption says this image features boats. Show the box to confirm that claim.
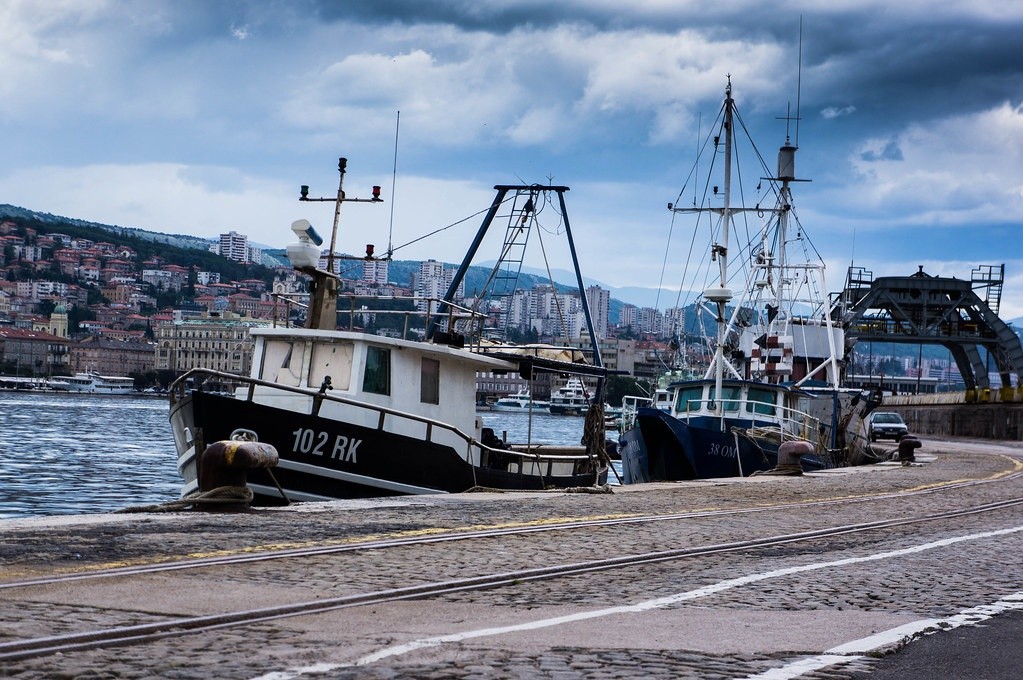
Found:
[579,306,718,433]
[615,12,884,486]
[164,108,634,508]
[550,375,597,415]
[486,380,550,413]
[44,357,135,394]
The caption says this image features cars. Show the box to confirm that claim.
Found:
[868,410,909,443]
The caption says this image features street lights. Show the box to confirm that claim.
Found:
[28,333,36,376]
